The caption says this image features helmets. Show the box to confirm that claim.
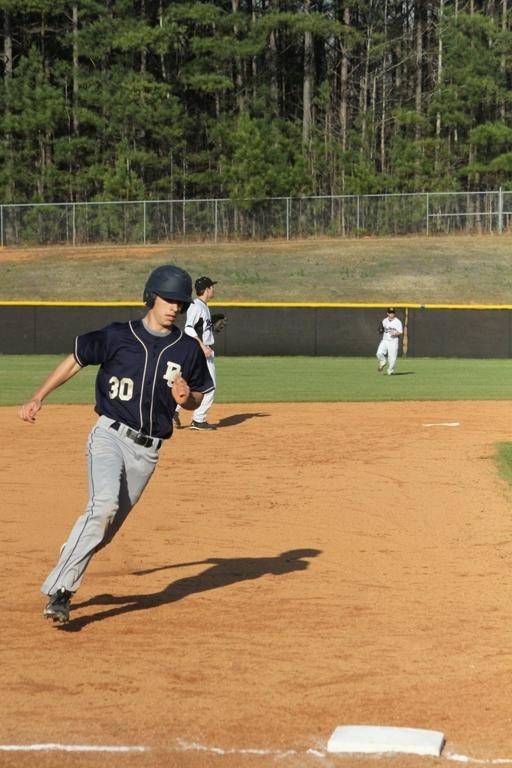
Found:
[142,265,195,314]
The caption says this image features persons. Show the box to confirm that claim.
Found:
[376,307,404,375]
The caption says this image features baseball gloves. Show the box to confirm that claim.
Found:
[211,313,228,335]
[376,320,384,333]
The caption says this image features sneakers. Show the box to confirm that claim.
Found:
[378,360,394,375]
[43,587,71,624]
[59,542,66,558]
[173,411,181,426]
[188,420,216,430]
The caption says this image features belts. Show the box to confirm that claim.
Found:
[111,422,162,450]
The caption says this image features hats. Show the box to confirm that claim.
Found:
[195,277,218,291]
[387,307,395,314]
